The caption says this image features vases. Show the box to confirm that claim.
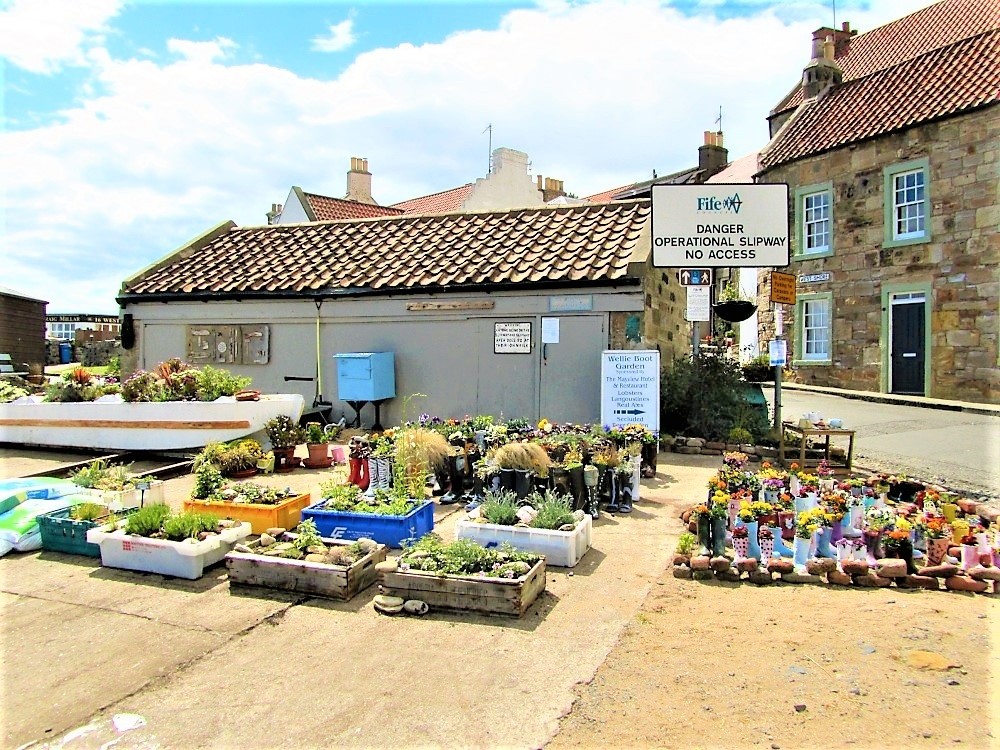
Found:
[688,460,1000,571]
[379,548,546,618]
[342,440,657,515]
[272,446,302,473]
[183,492,310,536]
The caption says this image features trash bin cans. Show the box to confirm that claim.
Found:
[58,343,71,364]
[728,383,769,422]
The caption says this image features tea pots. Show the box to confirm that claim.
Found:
[830,418,843,427]
[802,411,821,423]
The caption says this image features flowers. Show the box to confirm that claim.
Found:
[265,414,305,448]
[676,451,1000,546]
[394,527,537,579]
[350,413,656,453]
[191,465,291,507]
[50,358,251,403]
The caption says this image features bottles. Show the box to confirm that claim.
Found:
[818,419,825,427]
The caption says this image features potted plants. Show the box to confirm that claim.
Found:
[456,486,591,565]
[303,424,338,467]
[36,502,137,557]
[86,501,252,580]
[741,353,783,382]
[189,439,263,477]
[711,269,758,322]
[301,471,434,547]
[70,457,165,508]
[224,516,385,602]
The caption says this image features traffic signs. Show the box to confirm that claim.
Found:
[600,350,662,433]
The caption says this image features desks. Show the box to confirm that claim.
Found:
[782,421,855,475]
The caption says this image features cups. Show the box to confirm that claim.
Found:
[799,419,811,428]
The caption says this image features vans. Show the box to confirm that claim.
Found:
[0,354,13,372]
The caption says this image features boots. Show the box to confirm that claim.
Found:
[347,422,657,519]
[689,470,1000,575]
